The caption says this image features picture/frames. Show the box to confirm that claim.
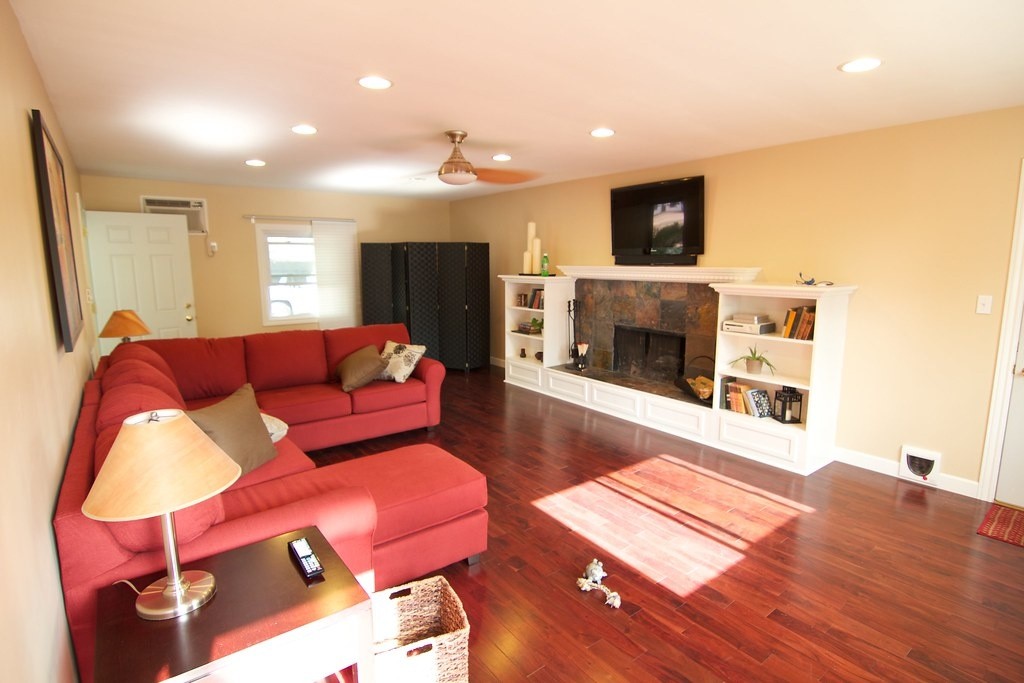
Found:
[30,108,85,353]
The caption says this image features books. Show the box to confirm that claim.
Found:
[517,288,544,310]
[780,305,817,340]
[721,376,774,418]
[519,322,541,334]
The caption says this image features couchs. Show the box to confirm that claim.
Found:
[53,323,489,683]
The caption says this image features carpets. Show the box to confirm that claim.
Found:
[977,505,1024,548]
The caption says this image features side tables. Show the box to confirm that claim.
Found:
[94,525,375,683]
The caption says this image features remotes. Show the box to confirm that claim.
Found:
[288,537,326,577]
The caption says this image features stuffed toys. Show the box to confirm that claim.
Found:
[576,559,621,609]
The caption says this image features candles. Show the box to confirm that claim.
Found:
[522,251,531,273]
[527,222,537,274]
[533,238,542,275]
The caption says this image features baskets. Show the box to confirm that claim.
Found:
[368,575,470,683]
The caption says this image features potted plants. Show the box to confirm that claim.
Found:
[498,264,858,481]
[729,345,778,376]
[531,318,544,338]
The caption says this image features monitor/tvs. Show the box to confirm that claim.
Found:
[611,174,706,265]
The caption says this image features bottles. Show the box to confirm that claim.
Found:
[541,253,549,277]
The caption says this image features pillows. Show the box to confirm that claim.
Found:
[185,383,279,479]
[373,339,427,383]
[334,345,389,392]
[259,413,289,444]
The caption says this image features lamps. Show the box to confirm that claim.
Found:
[77,408,241,620]
[772,386,802,424]
[99,311,151,344]
[437,129,478,185]
